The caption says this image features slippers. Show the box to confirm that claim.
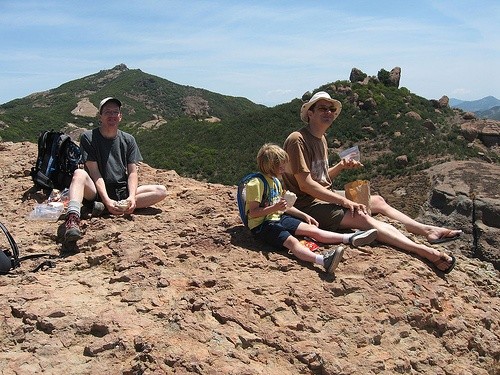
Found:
[430,251,455,274]
[429,228,463,243]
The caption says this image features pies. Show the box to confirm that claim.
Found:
[282,189,297,208]
[115,200,131,211]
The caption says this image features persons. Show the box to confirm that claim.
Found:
[65,97,167,242]
[280,91,464,274]
[244,143,378,274]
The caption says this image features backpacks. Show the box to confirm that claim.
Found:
[237,173,279,227]
[31,130,81,192]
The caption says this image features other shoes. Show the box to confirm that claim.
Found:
[323,247,344,274]
[350,228,378,248]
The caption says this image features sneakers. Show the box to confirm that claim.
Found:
[93,201,108,216]
[64,214,81,241]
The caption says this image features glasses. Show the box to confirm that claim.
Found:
[316,106,339,114]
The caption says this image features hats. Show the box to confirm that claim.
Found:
[99,97,122,112]
[300,91,342,126]
